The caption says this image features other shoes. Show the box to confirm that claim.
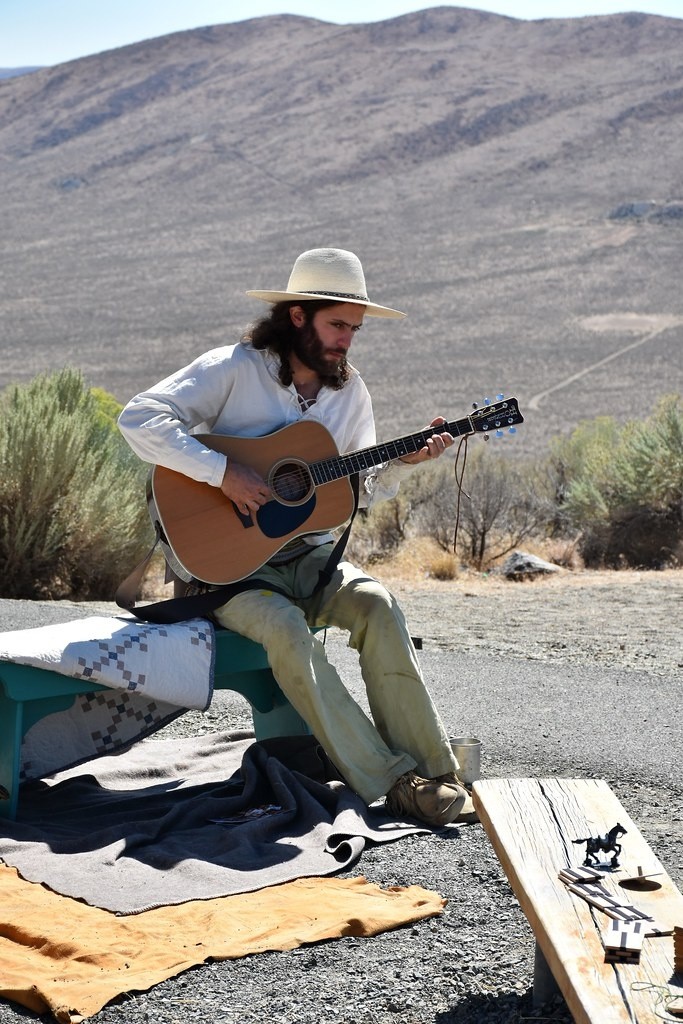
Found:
[384,771,468,827]
[434,772,480,822]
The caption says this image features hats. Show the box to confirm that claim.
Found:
[244,247,406,319]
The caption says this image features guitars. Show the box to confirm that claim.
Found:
[141,394,524,585]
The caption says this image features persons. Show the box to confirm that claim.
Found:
[117,248,483,825]
